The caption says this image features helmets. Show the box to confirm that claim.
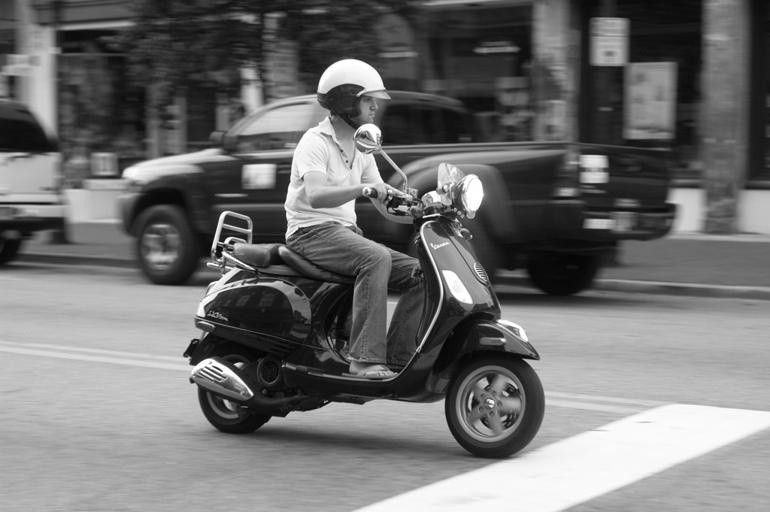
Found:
[317,59,391,118]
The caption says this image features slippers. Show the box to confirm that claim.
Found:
[342,364,398,379]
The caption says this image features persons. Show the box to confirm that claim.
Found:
[285,58,425,380]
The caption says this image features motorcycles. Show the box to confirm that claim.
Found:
[183,121,546,458]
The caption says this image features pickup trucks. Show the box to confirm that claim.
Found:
[117,86,679,285]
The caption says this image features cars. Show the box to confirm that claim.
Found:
[0,94,69,267]
